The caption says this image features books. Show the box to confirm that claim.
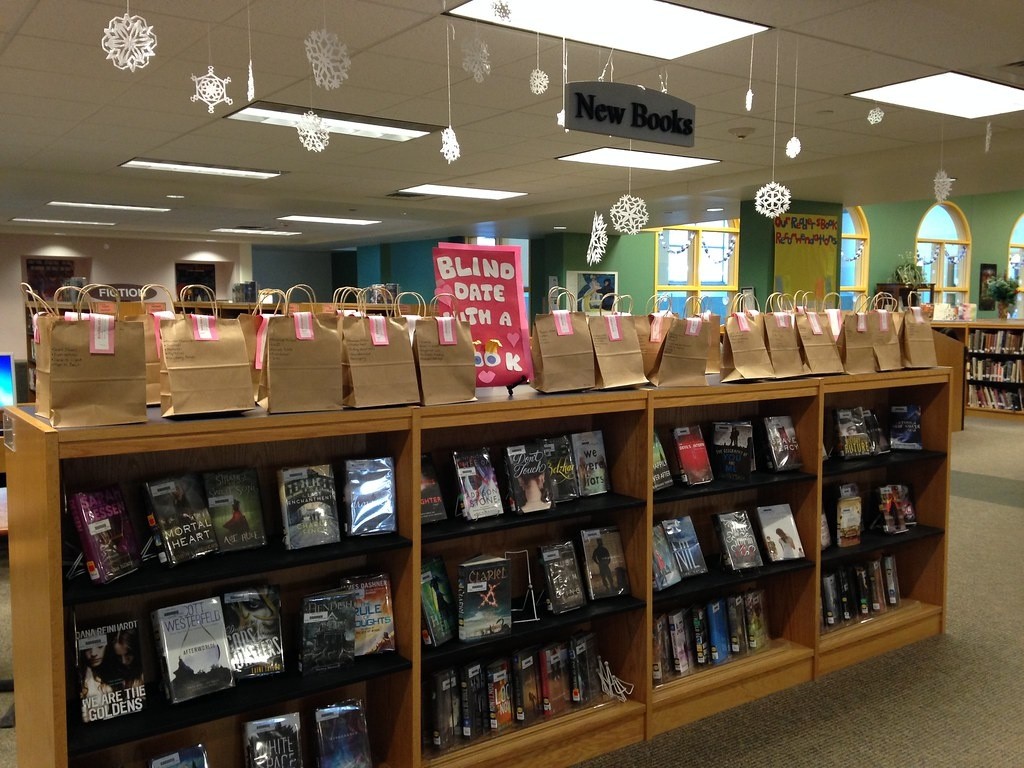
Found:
[922,302,977,323]
[823,405,923,461]
[74,615,144,723]
[821,482,916,549]
[821,553,898,626]
[420,452,447,525]
[651,502,806,591]
[967,329,1024,411]
[760,415,804,473]
[450,429,610,521]
[149,565,396,724]
[653,419,755,494]
[428,632,604,751]
[653,586,769,687]
[420,526,632,646]
[150,696,373,768]
[67,453,397,584]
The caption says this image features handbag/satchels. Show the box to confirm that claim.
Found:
[19,281,480,429]
[533,286,938,393]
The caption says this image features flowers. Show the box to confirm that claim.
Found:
[979,273,1020,306]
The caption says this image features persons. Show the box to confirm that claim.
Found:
[80,588,279,722]
[371,631,393,654]
[591,529,803,593]
[516,454,553,512]
[595,279,614,310]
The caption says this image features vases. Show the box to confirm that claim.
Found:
[997,300,1009,321]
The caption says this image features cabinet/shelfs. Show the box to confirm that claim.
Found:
[3,300,1024,768]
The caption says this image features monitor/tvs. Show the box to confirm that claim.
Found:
[0,352,17,410]
